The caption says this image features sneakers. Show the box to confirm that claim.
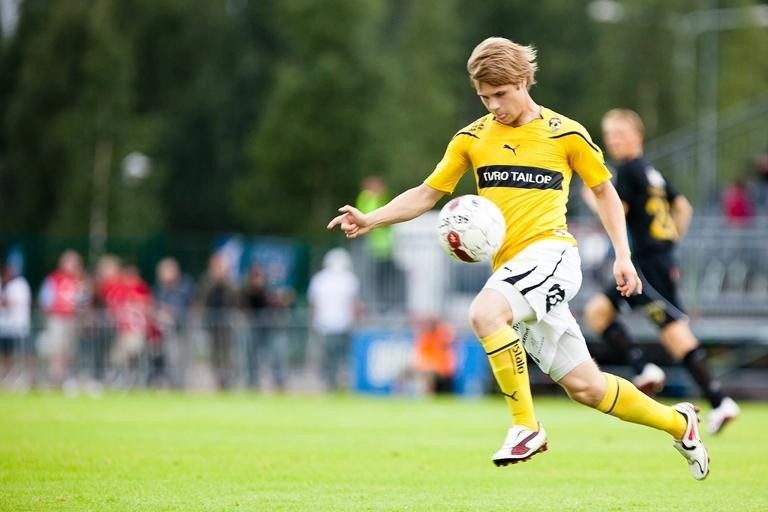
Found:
[491,421,549,467]
[670,403,710,480]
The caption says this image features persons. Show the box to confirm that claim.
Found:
[1,243,368,397]
[328,37,714,482]
[390,305,460,397]
[578,108,740,438]
[352,164,395,306]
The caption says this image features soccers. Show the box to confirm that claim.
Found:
[437,195,507,264]
